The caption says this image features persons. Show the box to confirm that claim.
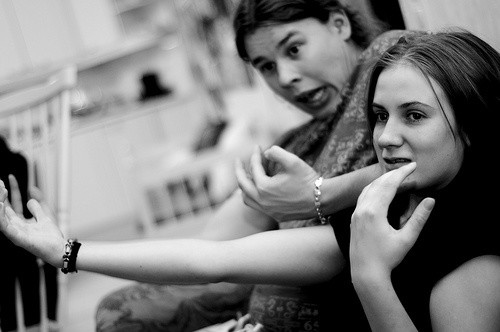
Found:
[0,27,500,331]
[90,0,430,330]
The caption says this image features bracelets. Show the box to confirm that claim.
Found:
[315,175,333,226]
[61,238,73,274]
[69,241,82,274]
